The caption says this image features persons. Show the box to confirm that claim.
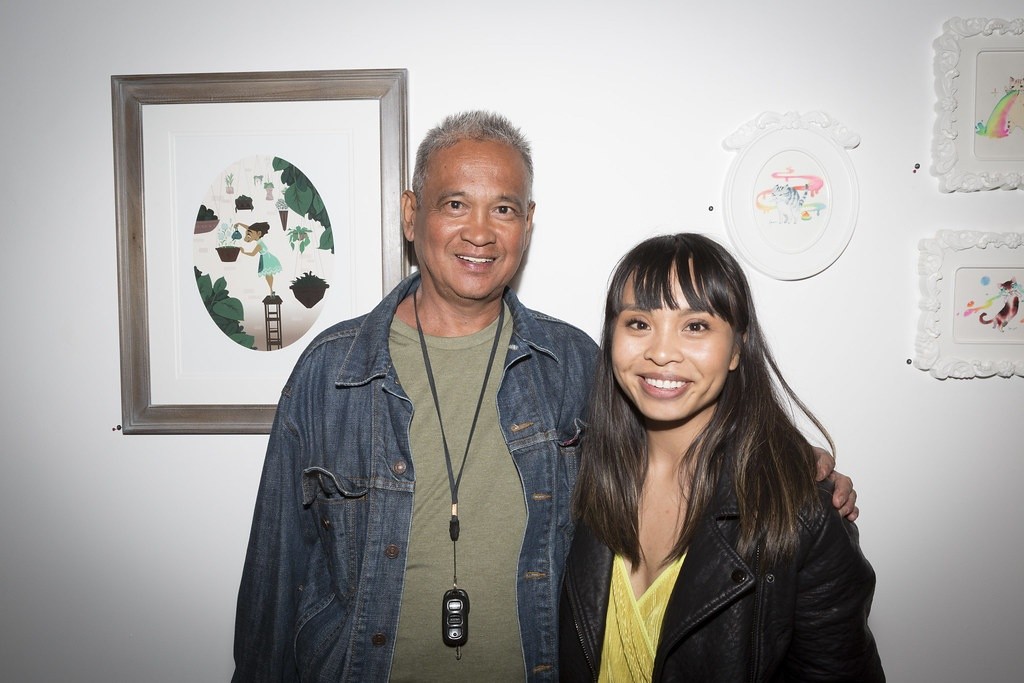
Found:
[232,115,859,683]
[556,232,886,683]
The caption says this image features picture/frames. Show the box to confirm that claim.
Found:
[930,17,1024,193]
[109,68,413,433]
[720,110,862,281]
[916,229,1024,378]
